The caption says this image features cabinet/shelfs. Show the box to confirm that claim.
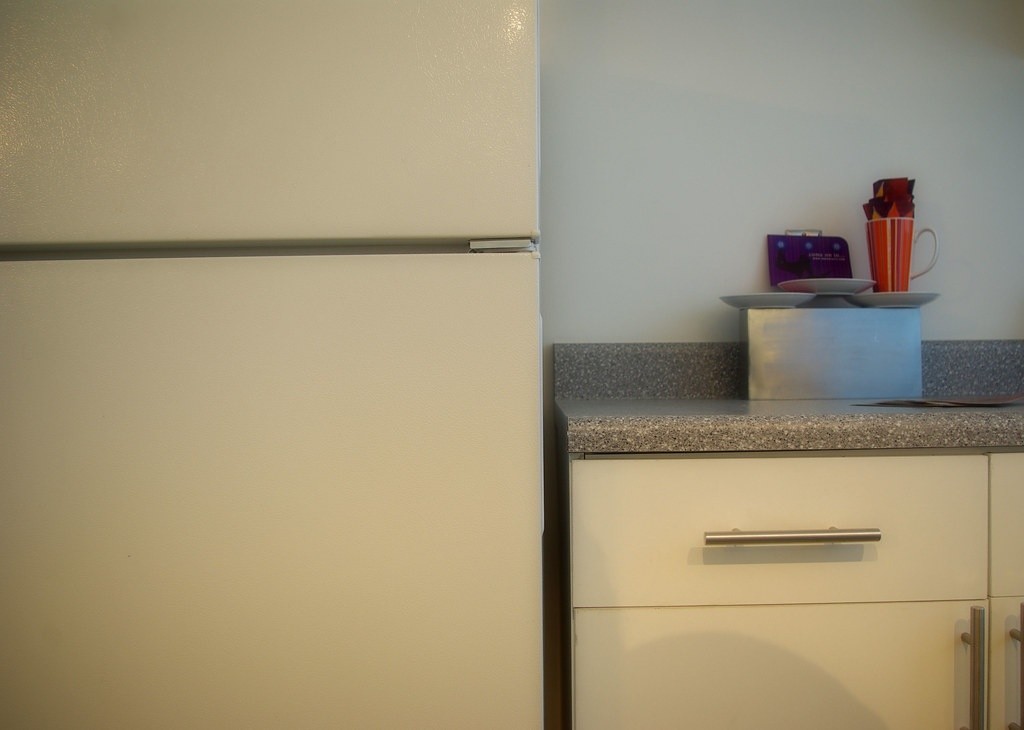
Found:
[570,451,1024,730]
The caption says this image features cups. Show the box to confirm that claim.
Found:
[865,218,939,293]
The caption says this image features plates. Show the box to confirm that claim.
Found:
[778,278,878,296]
[846,292,939,309]
[720,293,816,309]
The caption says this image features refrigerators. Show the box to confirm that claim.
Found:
[0,1,548,730]
